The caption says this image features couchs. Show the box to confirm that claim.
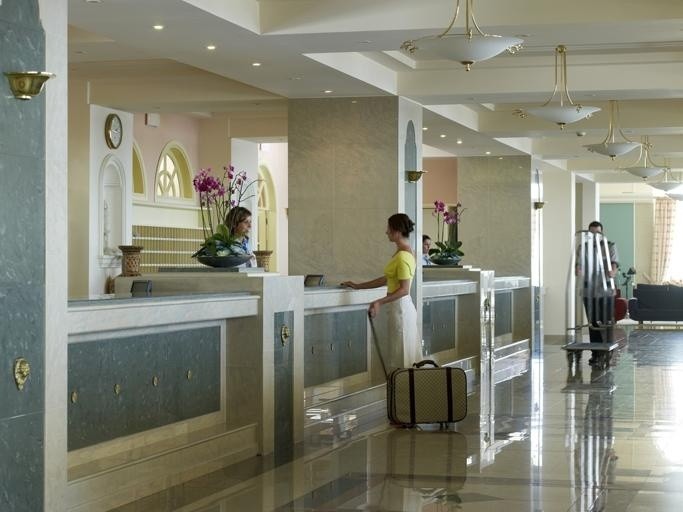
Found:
[628,283,683,325]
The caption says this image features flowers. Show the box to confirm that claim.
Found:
[192,164,264,238]
[430,198,467,240]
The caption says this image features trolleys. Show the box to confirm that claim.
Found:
[561,360,616,512]
[564,225,622,363]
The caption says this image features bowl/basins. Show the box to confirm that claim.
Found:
[199,255,252,270]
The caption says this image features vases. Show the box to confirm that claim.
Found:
[253,250,273,272]
[106,245,144,293]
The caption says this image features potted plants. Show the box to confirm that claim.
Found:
[189,223,254,268]
[428,240,465,265]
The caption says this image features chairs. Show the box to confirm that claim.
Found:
[613,287,627,323]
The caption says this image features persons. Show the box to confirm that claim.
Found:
[575,221,619,363]
[340,214,423,369]
[422,234,433,265]
[215,207,258,268]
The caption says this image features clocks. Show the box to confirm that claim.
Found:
[106,113,123,149]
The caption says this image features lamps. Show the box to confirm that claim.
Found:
[646,157,682,201]
[509,44,602,134]
[400,0,531,78]
[621,133,671,181]
[577,98,640,163]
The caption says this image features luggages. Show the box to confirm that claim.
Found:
[367,308,466,428]
